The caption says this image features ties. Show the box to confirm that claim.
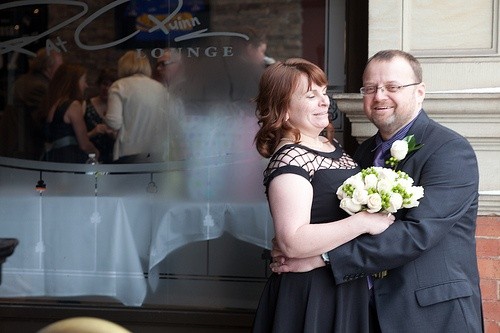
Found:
[373,147,384,167]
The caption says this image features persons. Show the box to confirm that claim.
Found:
[12,27,267,166]
[269,49,484,333]
[248,57,396,333]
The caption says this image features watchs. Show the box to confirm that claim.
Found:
[321,252,330,265]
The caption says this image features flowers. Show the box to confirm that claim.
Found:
[384,133,425,171]
[334,165,425,280]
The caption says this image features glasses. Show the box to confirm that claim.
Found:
[360,83,421,96]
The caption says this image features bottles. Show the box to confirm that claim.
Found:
[86,154,99,175]
